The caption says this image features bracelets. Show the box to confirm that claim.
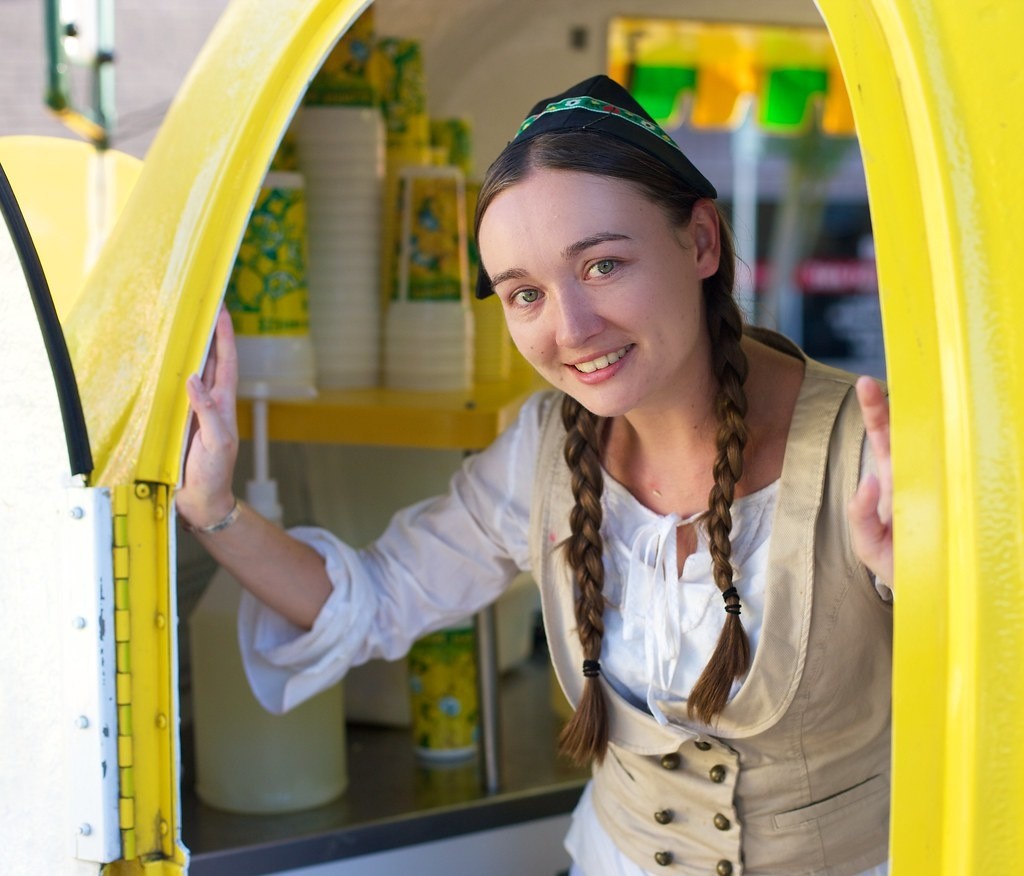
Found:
[183,497,242,532]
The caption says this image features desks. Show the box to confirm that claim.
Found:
[238,378,543,797]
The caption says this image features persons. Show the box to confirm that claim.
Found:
[177,75,893,876]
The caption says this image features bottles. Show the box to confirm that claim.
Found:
[187,380,348,814]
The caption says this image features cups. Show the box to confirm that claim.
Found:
[224,1,476,402]
[405,614,480,762]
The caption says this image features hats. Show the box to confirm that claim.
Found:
[475,75,718,300]
